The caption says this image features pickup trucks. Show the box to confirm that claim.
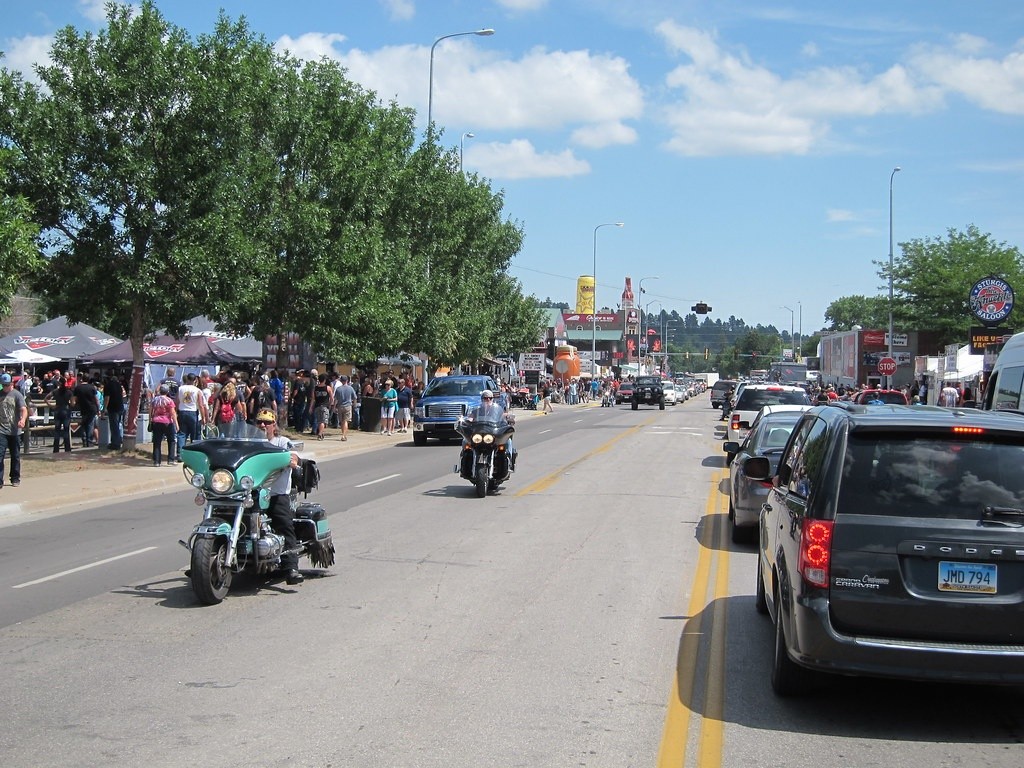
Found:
[412,374,509,446]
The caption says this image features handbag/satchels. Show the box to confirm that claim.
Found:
[148,421,152,432]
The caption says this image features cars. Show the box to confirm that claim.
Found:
[857,388,909,405]
[660,377,708,406]
[722,409,806,543]
[614,382,636,405]
[737,403,818,438]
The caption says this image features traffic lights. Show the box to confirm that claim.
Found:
[696,303,708,315]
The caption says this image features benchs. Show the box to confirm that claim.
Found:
[28,414,79,451]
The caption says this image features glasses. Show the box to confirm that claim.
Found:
[385,384,391,386]
[483,396,493,401]
[2,383,10,387]
[256,419,274,426]
[397,382,403,384]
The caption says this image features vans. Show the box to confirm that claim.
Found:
[963,331,1024,414]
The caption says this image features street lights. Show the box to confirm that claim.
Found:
[665,319,678,365]
[782,304,794,359]
[886,167,903,390]
[645,299,662,376]
[637,276,661,376]
[459,131,475,173]
[797,300,801,356]
[422,26,496,391]
[591,222,624,383]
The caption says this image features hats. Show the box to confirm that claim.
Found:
[0,374,11,383]
[340,375,348,381]
[23,371,28,376]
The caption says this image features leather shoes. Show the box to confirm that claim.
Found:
[285,569,304,586]
[184,569,191,578]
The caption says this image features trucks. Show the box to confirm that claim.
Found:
[695,372,720,388]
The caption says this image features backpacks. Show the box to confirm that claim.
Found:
[218,396,234,423]
[254,388,271,410]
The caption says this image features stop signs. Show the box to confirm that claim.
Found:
[878,357,897,376]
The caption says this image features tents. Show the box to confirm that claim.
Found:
[0,315,126,399]
[76,306,429,392]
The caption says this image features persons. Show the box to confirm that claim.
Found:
[459,390,516,473]
[0,365,132,489]
[890,378,928,406]
[251,407,304,585]
[806,380,882,406]
[540,374,636,412]
[140,365,426,466]
[939,380,972,408]
[448,363,530,411]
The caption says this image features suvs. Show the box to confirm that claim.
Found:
[722,385,809,466]
[710,379,737,410]
[742,400,1023,698]
[632,375,665,411]
[724,380,778,412]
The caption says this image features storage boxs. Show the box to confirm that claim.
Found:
[296,501,331,539]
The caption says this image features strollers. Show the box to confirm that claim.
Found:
[523,394,541,410]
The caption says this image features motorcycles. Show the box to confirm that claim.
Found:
[453,400,519,498]
[603,387,615,408]
[179,421,337,607]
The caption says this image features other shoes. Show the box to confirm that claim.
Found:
[13,479,21,486]
[107,443,121,450]
[168,458,178,465]
[176,454,183,462]
[152,461,160,466]
[381,426,408,436]
[83,442,94,447]
[0,482,4,489]
[294,426,346,441]
